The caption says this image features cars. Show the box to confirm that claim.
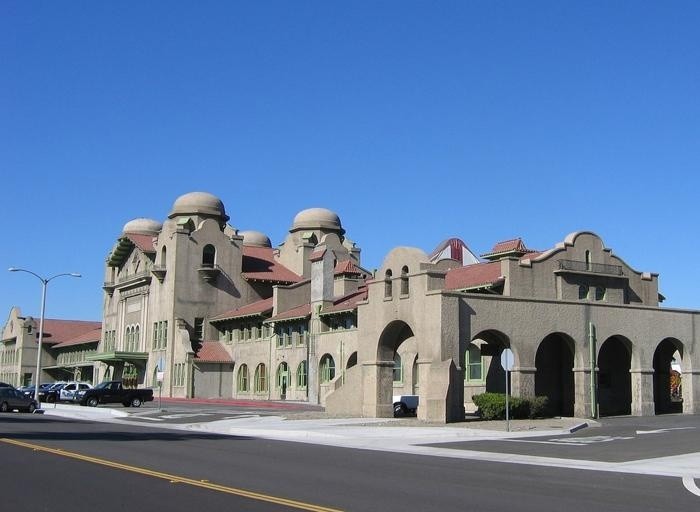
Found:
[17,382,93,404]
[0,381,38,414]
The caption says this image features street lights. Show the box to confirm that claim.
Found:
[10,267,82,406]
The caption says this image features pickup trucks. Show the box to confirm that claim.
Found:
[72,380,154,408]
